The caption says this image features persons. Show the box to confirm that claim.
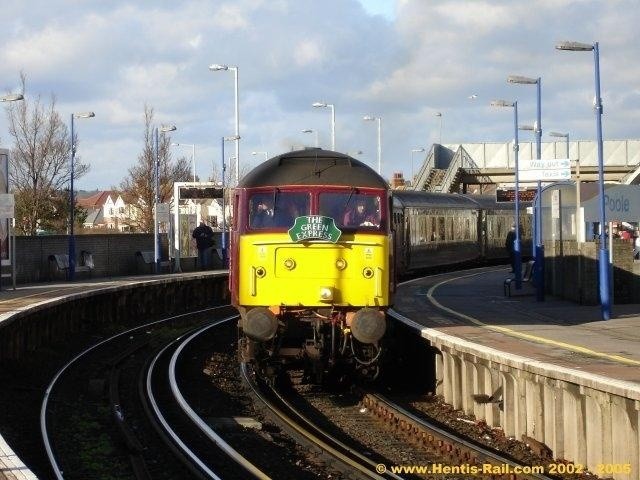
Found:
[288,200,303,219]
[343,197,378,226]
[253,198,285,227]
[192,219,216,269]
[505,222,523,274]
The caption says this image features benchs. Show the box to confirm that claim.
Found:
[48,253,93,281]
[504,260,536,298]
[137,250,172,274]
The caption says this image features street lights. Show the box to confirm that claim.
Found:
[490,95,570,295]
[216,133,270,272]
[151,124,178,275]
[66,110,97,281]
[1,90,25,106]
[294,99,448,190]
[552,36,619,324]
[208,63,242,187]
[504,74,550,304]
[167,140,198,188]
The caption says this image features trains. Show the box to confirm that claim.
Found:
[219,146,540,394]
